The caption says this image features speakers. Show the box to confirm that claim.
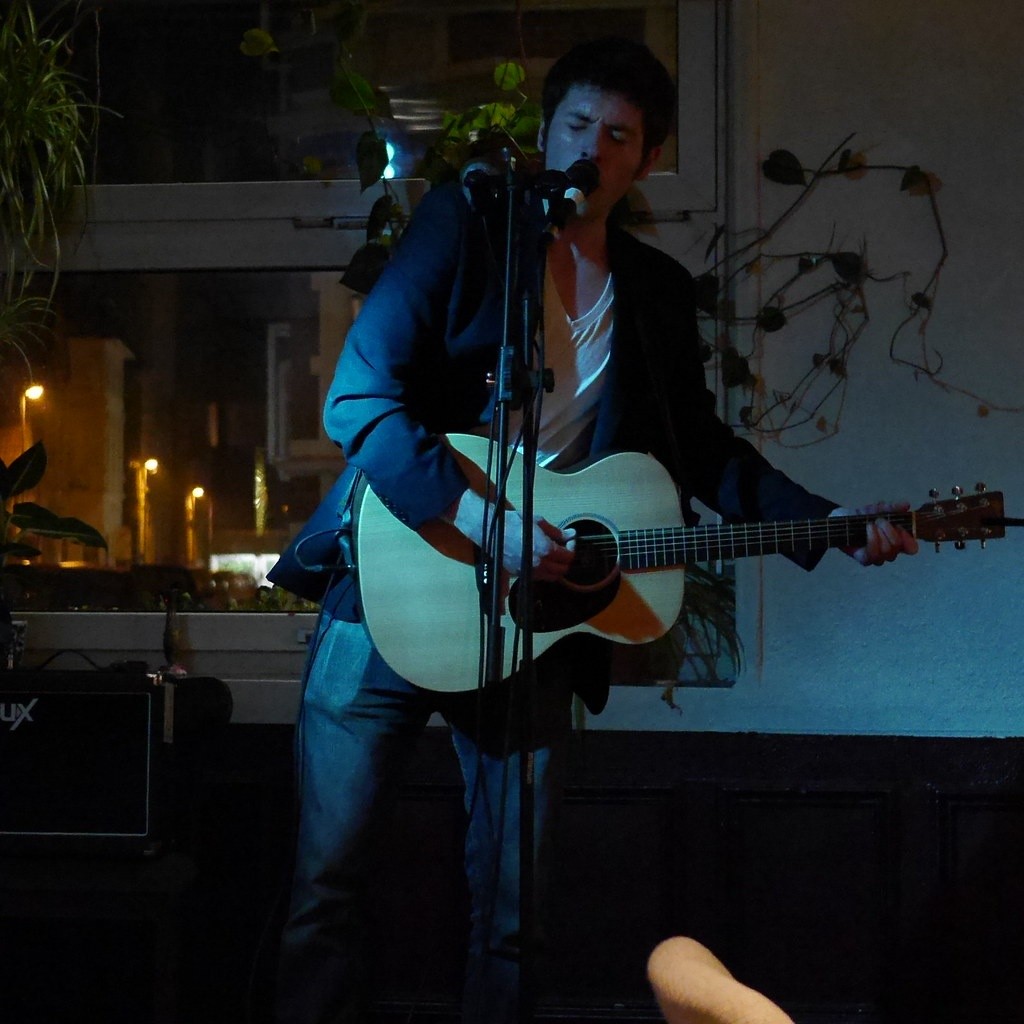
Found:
[0,668,163,839]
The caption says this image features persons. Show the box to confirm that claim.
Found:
[644,934,798,1024]
[265,36,918,1024]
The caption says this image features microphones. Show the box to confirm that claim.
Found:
[542,159,601,244]
[462,160,501,215]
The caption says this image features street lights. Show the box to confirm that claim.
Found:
[188,487,205,572]
[140,459,158,562]
[20,383,43,503]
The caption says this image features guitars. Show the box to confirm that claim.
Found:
[356,432,1007,694]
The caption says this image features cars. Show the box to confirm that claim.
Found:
[0,561,258,613]
